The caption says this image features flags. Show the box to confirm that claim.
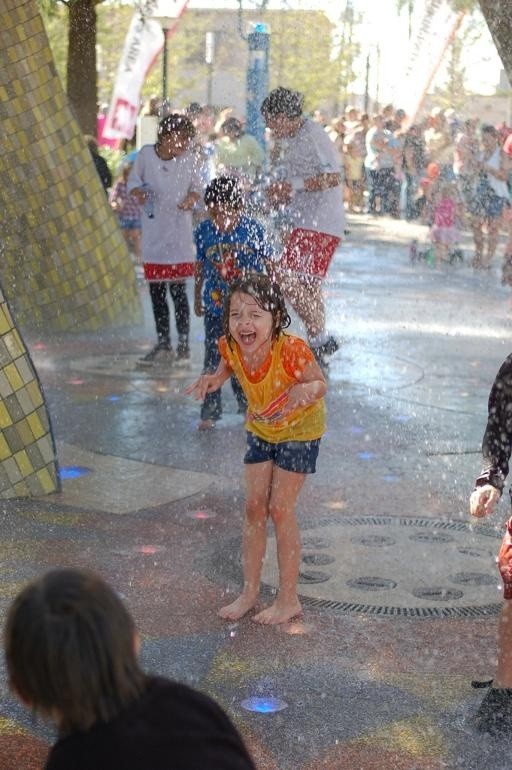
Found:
[97,0,188,149]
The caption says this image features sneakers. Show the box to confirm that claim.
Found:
[309,337,341,364]
[134,345,176,366]
[172,344,194,368]
[469,680,511,736]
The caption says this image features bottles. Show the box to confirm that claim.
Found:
[140,183,155,218]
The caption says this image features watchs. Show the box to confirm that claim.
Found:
[477,475,505,497]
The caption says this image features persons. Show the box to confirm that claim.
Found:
[186,274,328,627]
[128,114,203,370]
[193,177,277,433]
[260,87,345,360]
[83,97,512,286]
[471,354,511,734]
[5,569,256,769]
